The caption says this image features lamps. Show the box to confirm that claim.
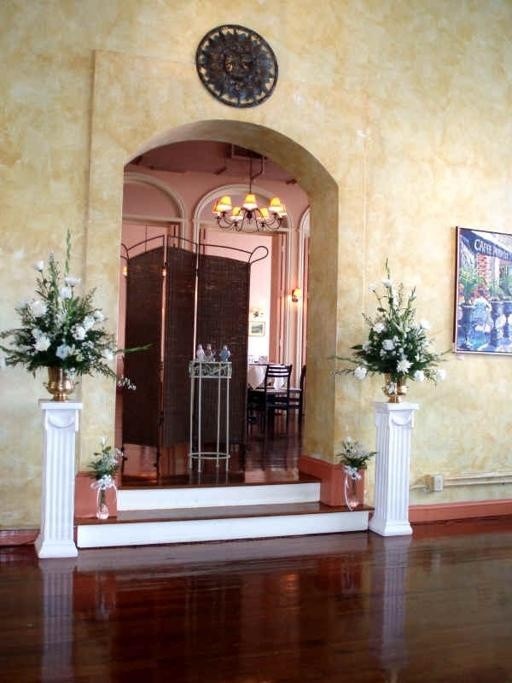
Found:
[213,151,289,235]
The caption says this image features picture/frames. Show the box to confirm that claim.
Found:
[449,224,511,358]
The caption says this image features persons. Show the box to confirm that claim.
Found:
[457,282,490,305]
[194,343,230,374]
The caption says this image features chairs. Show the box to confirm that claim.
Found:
[247,359,304,457]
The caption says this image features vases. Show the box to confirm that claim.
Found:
[98,500,109,521]
[384,372,409,402]
[45,364,74,398]
[349,487,359,507]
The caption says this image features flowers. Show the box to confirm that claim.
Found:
[337,436,373,504]
[328,253,451,395]
[0,223,155,398]
[83,435,127,517]
